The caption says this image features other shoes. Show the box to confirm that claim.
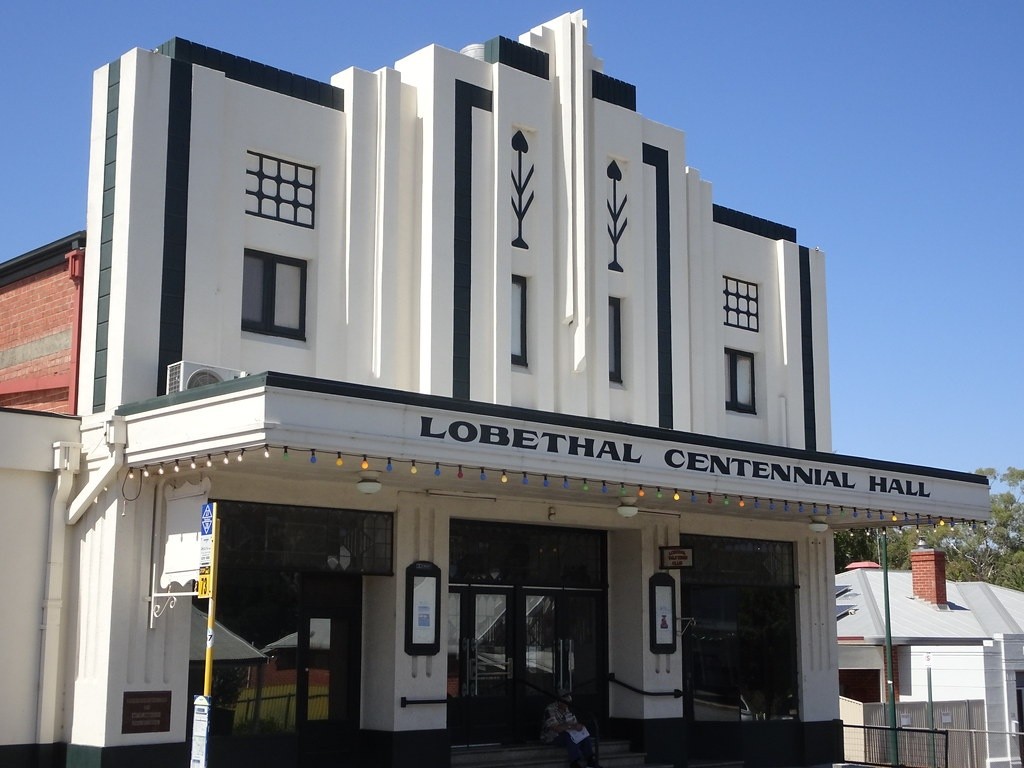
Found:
[587,762,603,768]
[570,763,582,768]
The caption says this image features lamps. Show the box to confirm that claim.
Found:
[617,497,638,518]
[357,470,383,494]
[808,515,828,532]
[166,360,247,395]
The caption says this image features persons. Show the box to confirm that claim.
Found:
[539,689,603,768]
